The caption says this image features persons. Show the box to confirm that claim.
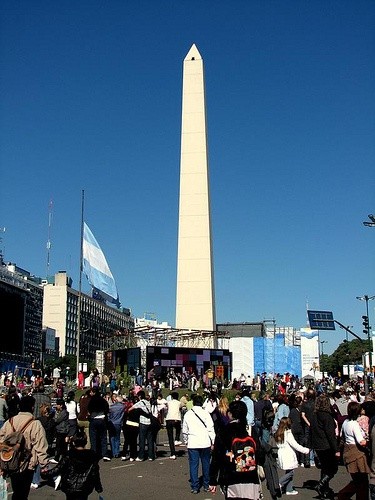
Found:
[0,366,375,500]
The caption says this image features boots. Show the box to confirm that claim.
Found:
[314,475,331,499]
[320,474,334,498]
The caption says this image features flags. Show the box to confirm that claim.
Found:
[82,222,118,300]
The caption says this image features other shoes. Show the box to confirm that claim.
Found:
[135,457,142,461]
[174,440,182,446]
[148,457,152,461]
[130,457,134,461]
[30,483,38,489]
[169,455,176,459]
[286,490,299,495]
[54,476,61,491]
[48,458,58,463]
[103,457,111,461]
[121,457,127,461]
[191,490,197,494]
[204,488,208,492]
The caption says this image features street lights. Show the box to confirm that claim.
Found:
[356,295,375,374]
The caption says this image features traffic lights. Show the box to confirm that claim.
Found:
[362,316,369,329]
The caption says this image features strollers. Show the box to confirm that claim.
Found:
[221,438,265,500]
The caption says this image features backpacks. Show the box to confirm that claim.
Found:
[0,417,39,474]
[226,425,256,473]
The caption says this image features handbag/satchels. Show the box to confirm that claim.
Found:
[150,416,161,433]
